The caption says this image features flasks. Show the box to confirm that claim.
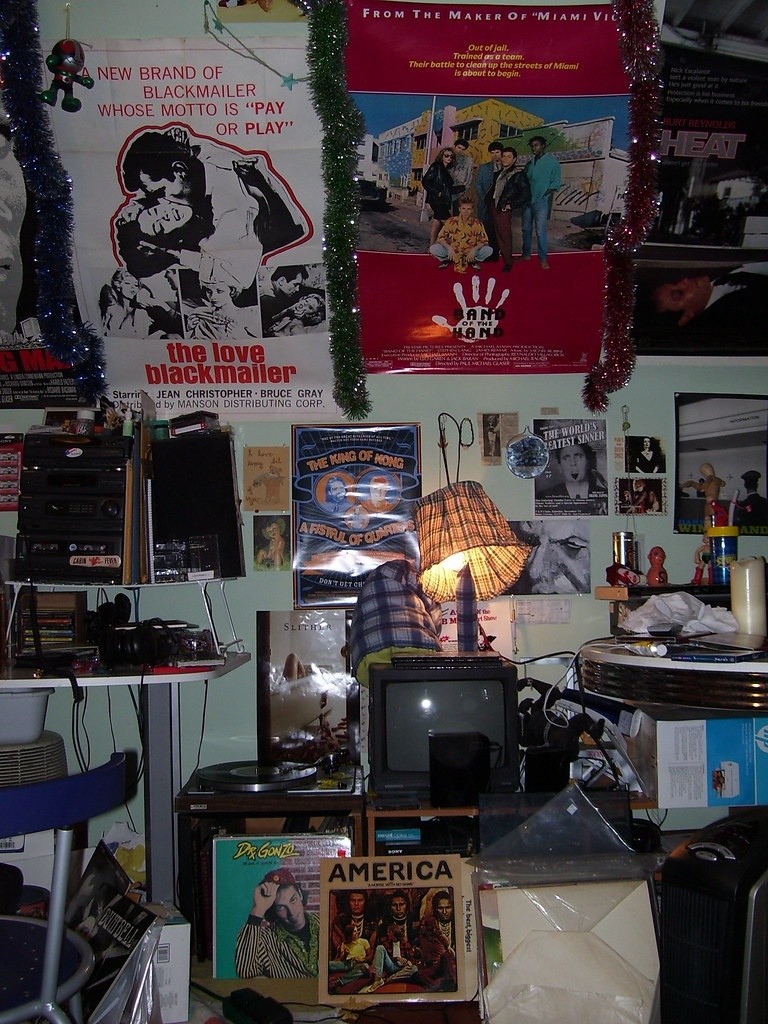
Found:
[613,531,635,572]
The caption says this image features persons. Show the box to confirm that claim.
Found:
[422,136,560,275]
[235,867,320,979]
[637,262,768,348]
[607,463,728,587]
[535,436,606,516]
[733,470,768,536]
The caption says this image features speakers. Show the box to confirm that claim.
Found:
[427,728,490,808]
[151,432,246,579]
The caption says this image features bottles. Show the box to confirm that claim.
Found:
[153,420,169,439]
[122,407,133,436]
[75,411,95,435]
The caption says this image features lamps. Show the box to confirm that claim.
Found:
[410,412,532,653]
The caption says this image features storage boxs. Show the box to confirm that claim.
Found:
[19,591,87,651]
[478,879,660,986]
[625,707,768,807]
[142,902,191,1022]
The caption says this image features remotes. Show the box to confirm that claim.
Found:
[648,622,683,635]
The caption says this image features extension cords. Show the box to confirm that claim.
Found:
[222,996,258,1024]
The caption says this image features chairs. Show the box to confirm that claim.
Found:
[0,751,136,1024]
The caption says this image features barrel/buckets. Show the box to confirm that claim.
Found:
[0,687,55,744]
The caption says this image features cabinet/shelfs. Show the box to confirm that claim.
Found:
[175,760,656,962]
[4,577,246,657]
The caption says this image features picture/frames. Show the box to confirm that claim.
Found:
[673,392,768,536]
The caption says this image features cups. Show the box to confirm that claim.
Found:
[708,526,738,585]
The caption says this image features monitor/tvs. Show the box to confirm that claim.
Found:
[368,665,521,795]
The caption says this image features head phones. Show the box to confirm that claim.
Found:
[110,618,173,666]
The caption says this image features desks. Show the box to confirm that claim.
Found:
[0,653,252,905]
